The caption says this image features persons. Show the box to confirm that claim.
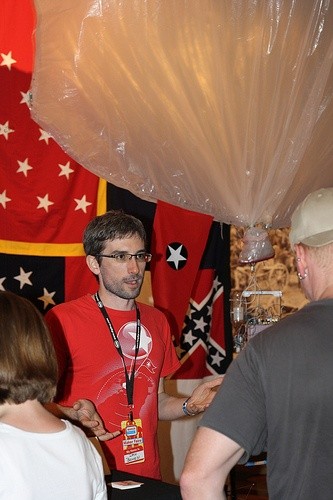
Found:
[179,187,333,500]
[0,290,107,500]
[41,212,223,483]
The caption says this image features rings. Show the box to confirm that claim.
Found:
[95,436,100,440]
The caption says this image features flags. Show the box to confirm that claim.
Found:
[0,0,233,377]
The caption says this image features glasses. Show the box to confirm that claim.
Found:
[94,253,152,263]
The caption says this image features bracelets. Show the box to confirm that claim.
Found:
[183,398,195,416]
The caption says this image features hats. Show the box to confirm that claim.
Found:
[289,187,332,252]
